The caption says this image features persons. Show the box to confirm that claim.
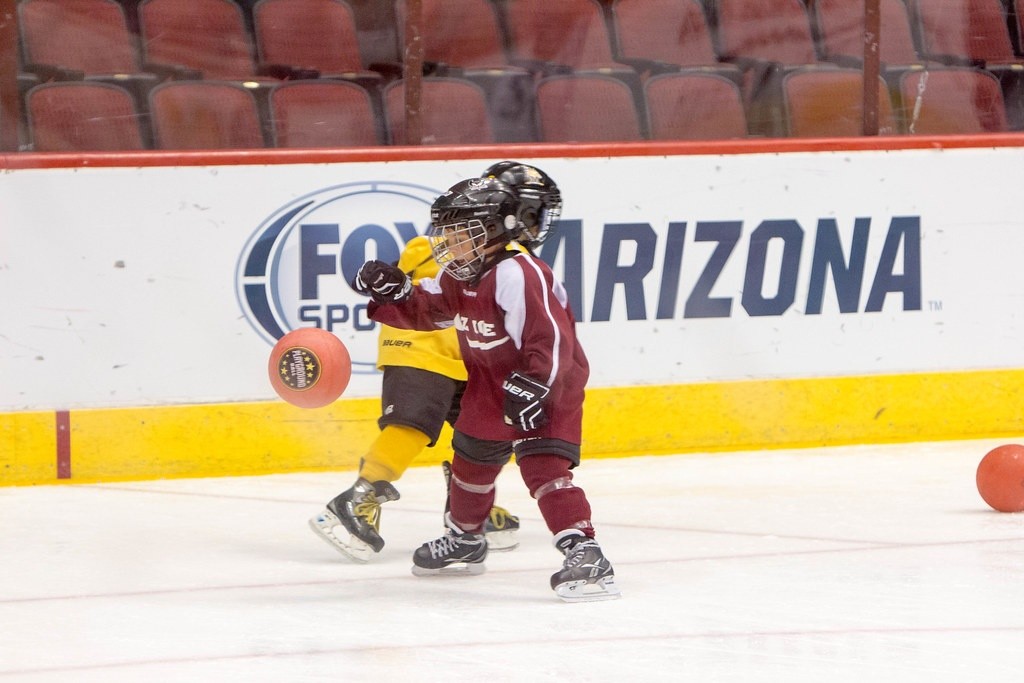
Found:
[353,179,619,599]
[311,161,559,562]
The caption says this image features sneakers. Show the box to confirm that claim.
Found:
[412,511,488,575]
[550,527,621,602]
[442,460,519,548]
[309,457,399,561]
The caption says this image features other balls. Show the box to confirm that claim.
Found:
[268,326,353,410]
[975,444,1024,514]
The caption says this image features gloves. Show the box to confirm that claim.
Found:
[353,259,412,303]
[504,370,551,429]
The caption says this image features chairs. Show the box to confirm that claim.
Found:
[252,1,408,82]
[23,80,144,151]
[145,82,266,152]
[897,0,1024,132]
[532,72,646,142]
[17,0,184,82]
[610,0,783,79]
[709,0,887,75]
[782,66,901,138]
[641,72,750,140]
[267,78,379,147]
[380,76,501,145]
[812,0,963,75]
[396,1,609,77]
[137,0,293,81]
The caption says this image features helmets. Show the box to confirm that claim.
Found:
[428,177,521,286]
[481,160,562,248]
